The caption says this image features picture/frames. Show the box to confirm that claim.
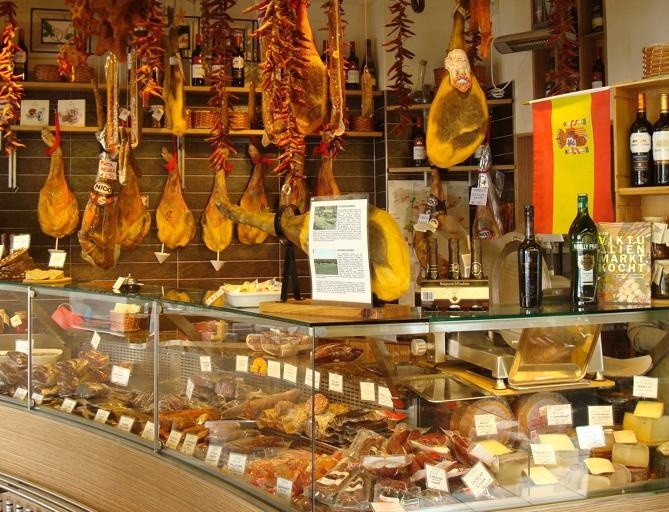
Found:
[163,13,198,60]
[29,8,92,56]
[199,16,255,64]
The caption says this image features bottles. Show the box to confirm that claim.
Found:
[544,57,554,96]
[361,39,376,91]
[652,93,669,186]
[413,115,425,166]
[191,34,205,86]
[320,40,329,65]
[517,204,543,308]
[591,46,604,88]
[231,36,244,87]
[568,193,599,306]
[629,92,653,186]
[14,29,28,81]
[345,41,359,90]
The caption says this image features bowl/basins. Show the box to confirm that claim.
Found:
[31,349,63,364]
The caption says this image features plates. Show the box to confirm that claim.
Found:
[328,349,364,364]
[398,373,495,403]
[397,365,425,376]
[221,282,282,307]
[508,325,602,385]
[23,278,72,284]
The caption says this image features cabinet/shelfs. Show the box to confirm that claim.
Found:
[612,75,669,304]
[529,0,607,101]
[0,284,669,512]
[1,79,384,137]
[386,97,515,174]
